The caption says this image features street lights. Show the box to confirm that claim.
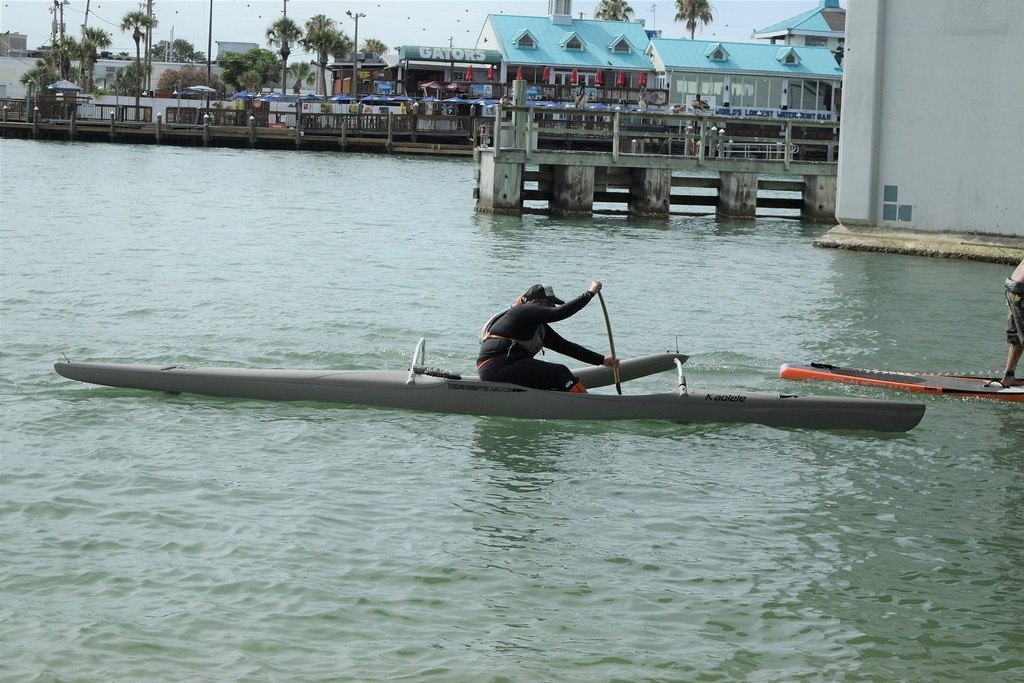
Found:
[345,10,366,103]
[54,0,70,78]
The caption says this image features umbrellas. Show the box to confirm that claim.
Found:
[570,68,577,84]
[360,95,413,101]
[639,71,646,88]
[226,91,254,101]
[466,66,475,82]
[543,66,550,84]
[329,94,355,101]
[179,89,199,95]
[525,100,586,108]
[595,69,603,85]
[423,96,466,116]
[617,70,625,87]
[516,66,524,80]
[189,85,217,107]
[470,96,511,117]
[487,64,495,82]
[300,94,323,100]
[594,102,654,111]
[256,93,289,102]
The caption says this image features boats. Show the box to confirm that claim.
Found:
[780,361,1024,403]
[54,338,927,433]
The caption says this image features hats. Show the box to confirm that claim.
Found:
[523,284,565,304]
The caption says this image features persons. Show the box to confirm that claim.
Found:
[477,281,620,394]
[999,258,1024,386]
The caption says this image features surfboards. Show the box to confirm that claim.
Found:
[778,361,1024,402]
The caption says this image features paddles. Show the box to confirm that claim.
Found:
[597,288,621,395]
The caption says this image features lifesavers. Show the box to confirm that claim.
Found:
[670,103,686,112]
